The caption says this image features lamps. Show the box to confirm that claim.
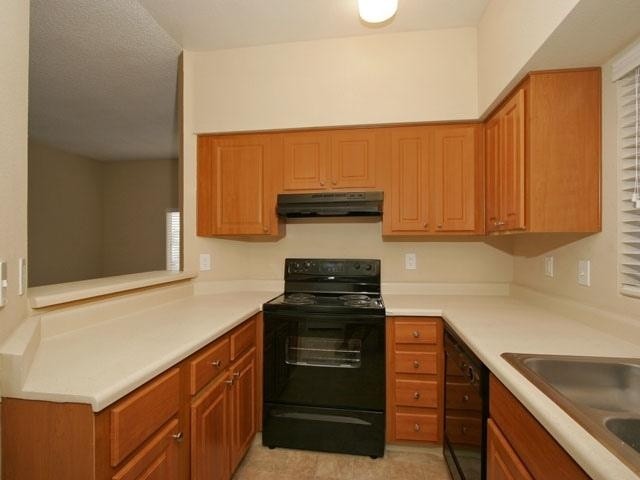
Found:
[356,0,399,24]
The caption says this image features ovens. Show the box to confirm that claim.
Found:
[262,316,385,458]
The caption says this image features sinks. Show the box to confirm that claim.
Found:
[602,414,639,457]
[517,356,640,414]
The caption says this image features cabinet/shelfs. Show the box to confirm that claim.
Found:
[1,363,191,479]
[386,317,445,448]
[194,131,286,238]
[276,125,387,193]
[183,313,264,480]
[478,65,603,236]
[485,372,591,480]
[382,118,480,237]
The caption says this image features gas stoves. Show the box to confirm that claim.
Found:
[262,291,385,314]
